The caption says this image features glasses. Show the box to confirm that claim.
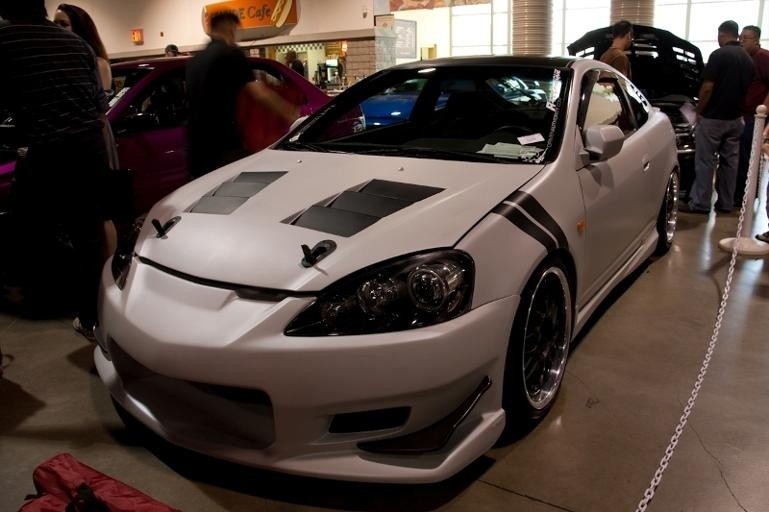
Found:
[739,35,759,42]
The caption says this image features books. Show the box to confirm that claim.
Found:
[679,100,699,128]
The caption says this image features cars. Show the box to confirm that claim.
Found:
[568,26,719,202]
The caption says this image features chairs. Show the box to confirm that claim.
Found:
[424,88,490,144]
[532,88,622,158]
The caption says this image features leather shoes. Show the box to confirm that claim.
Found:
[713,201,733,213]
[677,201,711,215]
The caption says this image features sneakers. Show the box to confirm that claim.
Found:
[72,317,96,340]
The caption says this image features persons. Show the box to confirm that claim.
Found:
[183,9,314,178]
[599,20,634,81]
[674,17,755,217]
[164,44,178,57]
[731,25,769,209]
[1,1,118,342]
[52,3,123,267]
[285,50,304,78]
